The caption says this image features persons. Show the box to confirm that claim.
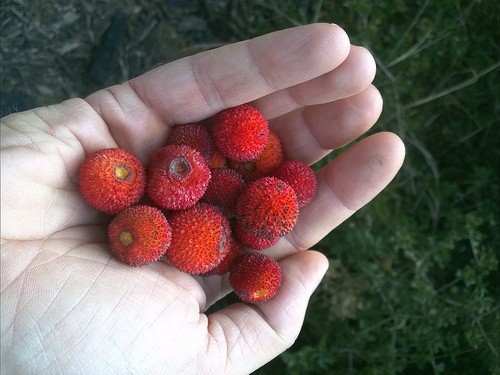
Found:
[0,19,406,375]
